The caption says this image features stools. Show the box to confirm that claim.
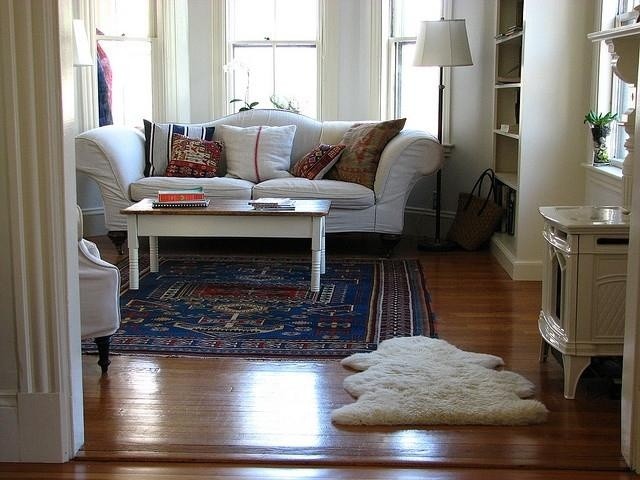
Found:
[537,206,632,400]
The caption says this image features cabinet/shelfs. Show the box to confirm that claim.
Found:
[490,0,595,281]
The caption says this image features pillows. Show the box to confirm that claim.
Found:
[142,120,215,177]
[220,123,297,184]
[290,143,347,180]
[322,118,407,191]
[165,132,224,178]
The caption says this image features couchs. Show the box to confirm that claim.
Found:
[77,204,122,377]
[75,108,446,260]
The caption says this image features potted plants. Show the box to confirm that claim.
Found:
[583,109,620,167]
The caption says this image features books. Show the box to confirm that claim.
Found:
[152,187,211,208]
[248,197,295,211]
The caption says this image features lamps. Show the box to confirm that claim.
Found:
[413,16,474,253]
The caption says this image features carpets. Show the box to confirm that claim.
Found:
[331,335,552,426]
[82,252,440,362]
